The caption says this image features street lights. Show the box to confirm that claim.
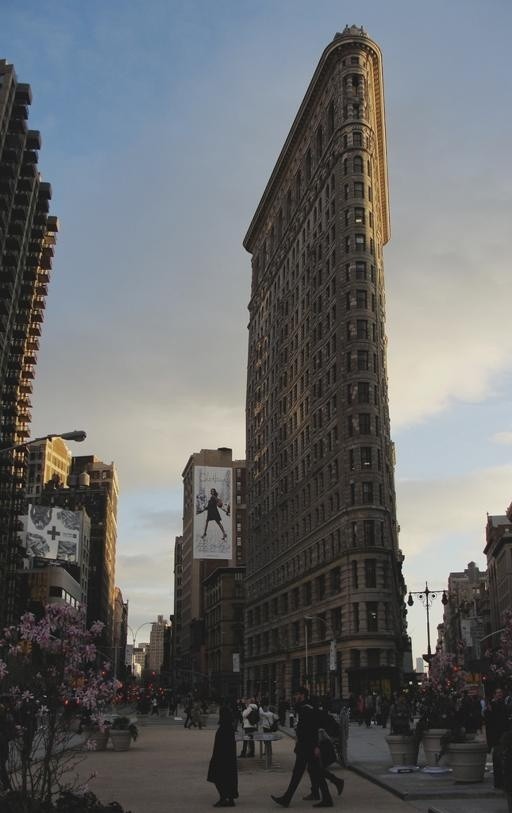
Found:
[406,580,450,681]
[302,613,338,699]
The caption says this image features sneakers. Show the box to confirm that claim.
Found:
[338,780,344,796]
[271,795,290,807]
[215,798,236,807]
[302,792,334,808]
[239,751,255,758]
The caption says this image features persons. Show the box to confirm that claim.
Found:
[20,691,38,759]
[200,489,229,540]
[1,704,13,792]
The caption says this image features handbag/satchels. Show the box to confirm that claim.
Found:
[248,709,260,725]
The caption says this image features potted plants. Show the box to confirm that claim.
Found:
[381,695,489,787]
[81,713,142,754]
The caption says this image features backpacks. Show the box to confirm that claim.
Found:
[268,716,280,732]
[317,727,337,769]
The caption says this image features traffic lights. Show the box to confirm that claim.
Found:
[152,671,157,679]
[481,674,486,683]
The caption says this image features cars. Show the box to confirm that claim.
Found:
[64,689,86,713]
